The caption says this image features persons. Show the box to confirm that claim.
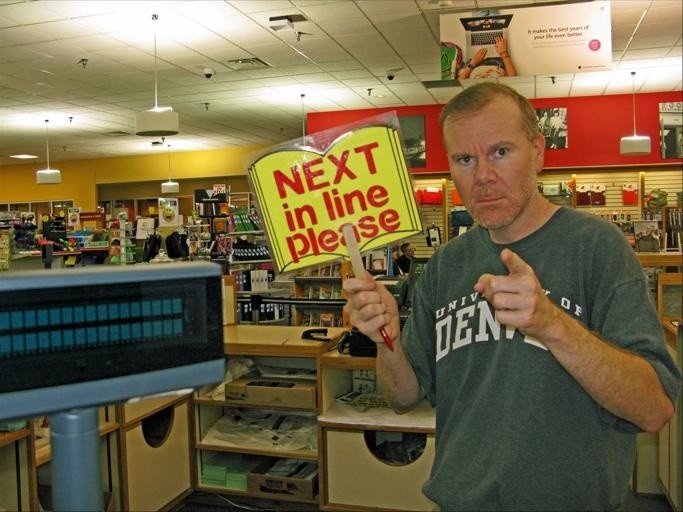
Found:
[393,242,415,275]
[480,19,492,30]
[343,87,682,511]
[456,37,516,79]
[534,108,563,150]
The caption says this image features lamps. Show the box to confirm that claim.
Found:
[134,14,179,137]
[34,119,61,185]
[619,72,652,156]
[160,145,180,194]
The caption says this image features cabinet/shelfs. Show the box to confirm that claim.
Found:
[319,352,440,510]
[108,219,392,327]
[193,328,350,511]
[100,386,195,511]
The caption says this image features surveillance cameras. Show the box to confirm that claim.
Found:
[387,71,395,82]
[202,69,216,80]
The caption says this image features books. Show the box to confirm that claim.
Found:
[226,212,388,327]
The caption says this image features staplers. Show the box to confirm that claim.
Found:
[301,328,331,341]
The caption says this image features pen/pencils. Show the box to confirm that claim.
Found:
[379,326,395,354]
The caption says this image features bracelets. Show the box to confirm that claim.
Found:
[467,62,473,69]
[499,52,509,57]
